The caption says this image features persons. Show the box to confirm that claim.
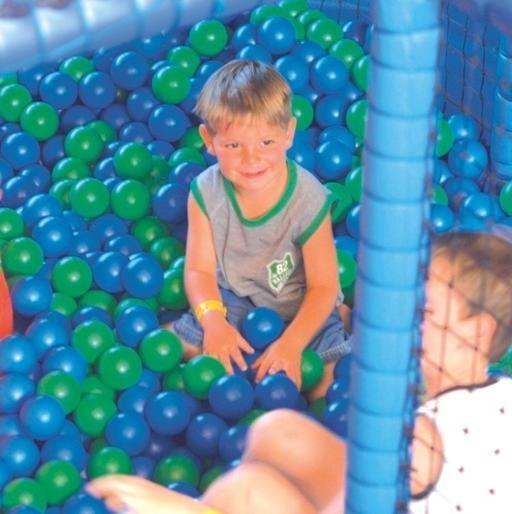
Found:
[157,58,348,400]
[199,232,512,514]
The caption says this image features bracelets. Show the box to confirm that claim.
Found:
[195,300,225,320]
